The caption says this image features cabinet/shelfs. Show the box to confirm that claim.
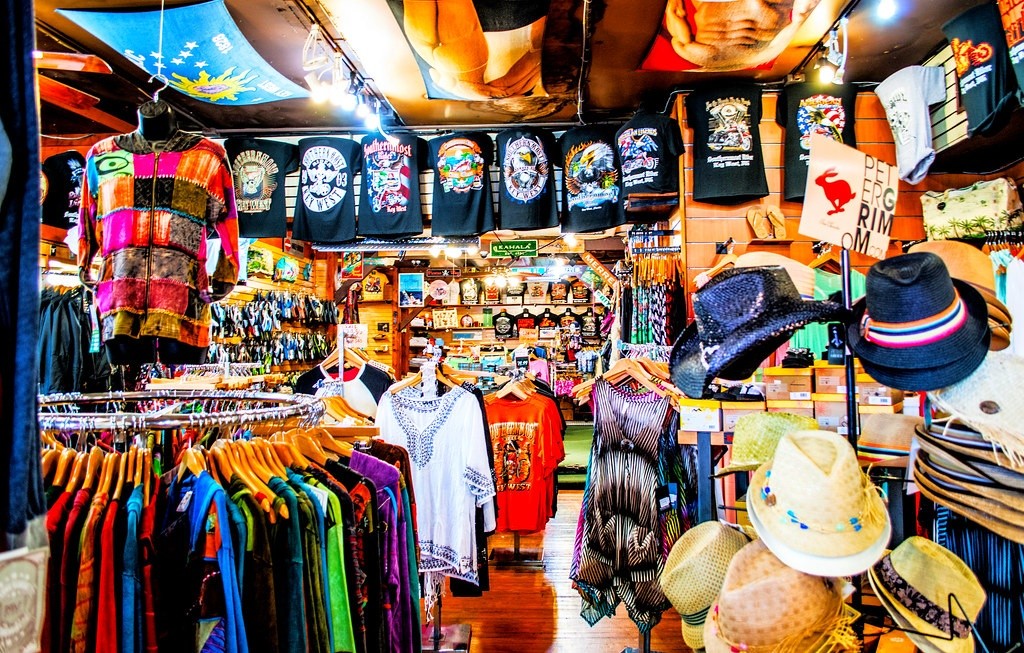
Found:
[391,268,616,423]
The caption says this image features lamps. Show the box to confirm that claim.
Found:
[782,2,898,92]
[290,0,394,131]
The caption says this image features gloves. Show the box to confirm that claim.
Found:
[203,289,339,366]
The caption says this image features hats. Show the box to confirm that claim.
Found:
[857,413,926,467]
[847,251,990,391]
[668,264,846,400]
[703,538,851,653]
[911,349,1024,547]
[747,431,893,577]
[868,536,987,653]
[715,411,819,478]
[661,520,762,649]
[908,241,1012,351]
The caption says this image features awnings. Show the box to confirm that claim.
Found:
[52,0,315,108]
[634,0,818,75]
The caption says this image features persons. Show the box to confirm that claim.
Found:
[401,0,549,99]
[78,100,238,367]
